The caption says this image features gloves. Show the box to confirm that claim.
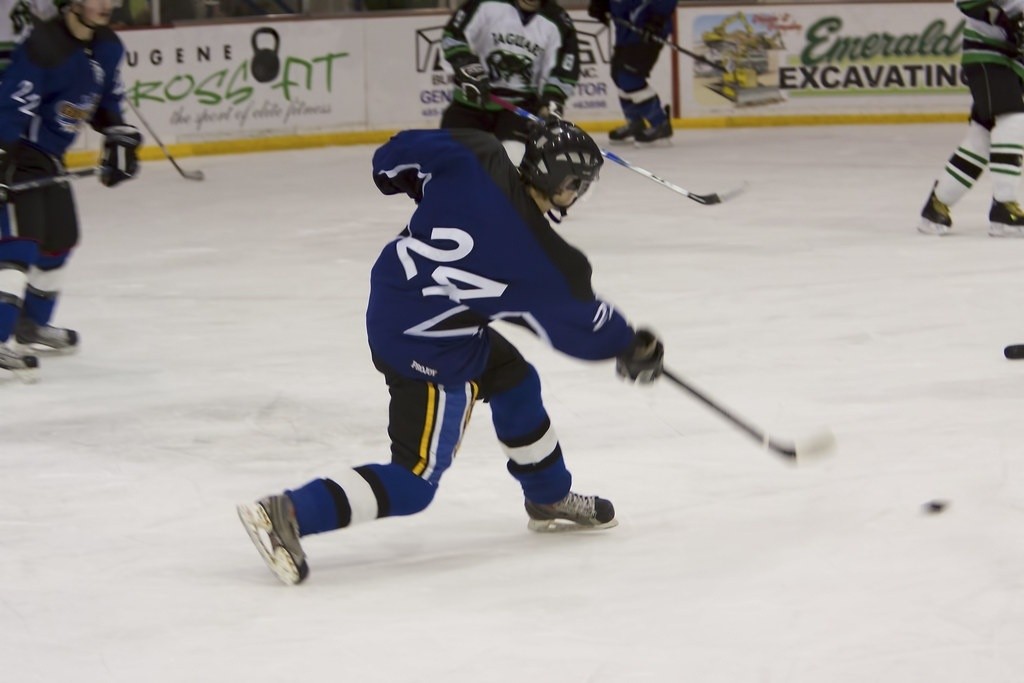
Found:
[538,84,567,122]
[98,123,143,187]
[588,0,614,27]
[616,330,664,385]
[453,53,491,108]
[997,11,1024,48]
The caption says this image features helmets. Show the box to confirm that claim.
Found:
[520,120,603,214]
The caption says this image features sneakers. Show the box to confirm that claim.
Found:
[526,490,619,534]
[236,492,309,587]
[606,113,637,146]
[915,180,951,236]
[0,343,38,371]
[16,316,80,352]
[632,102,673,146]
[985,196,1024,237]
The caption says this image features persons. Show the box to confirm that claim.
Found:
[257,120,666,588]
[586,0,679,142]
[435,0,579,152]
[0,0,143,371]
[920,0,1024,228]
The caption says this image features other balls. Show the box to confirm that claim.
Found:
[928,503,944,511]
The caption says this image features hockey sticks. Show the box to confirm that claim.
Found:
[662,366,839,461]
[606,9,732,75]
[1004,344,1024,361]
[486,92,743,205]
[123,92,204,180]
